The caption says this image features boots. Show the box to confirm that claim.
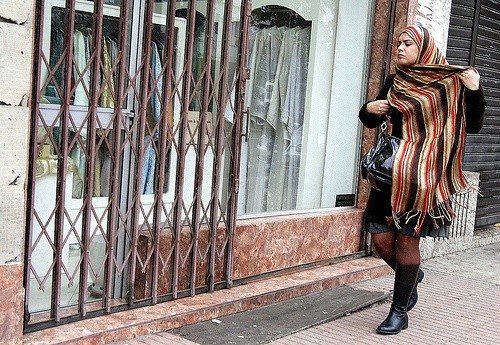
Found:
[375,258,420,335]
[382,255,424,312]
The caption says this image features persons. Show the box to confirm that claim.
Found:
[358,25,487,334]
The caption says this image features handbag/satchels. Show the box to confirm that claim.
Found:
[360,130,400,192]
